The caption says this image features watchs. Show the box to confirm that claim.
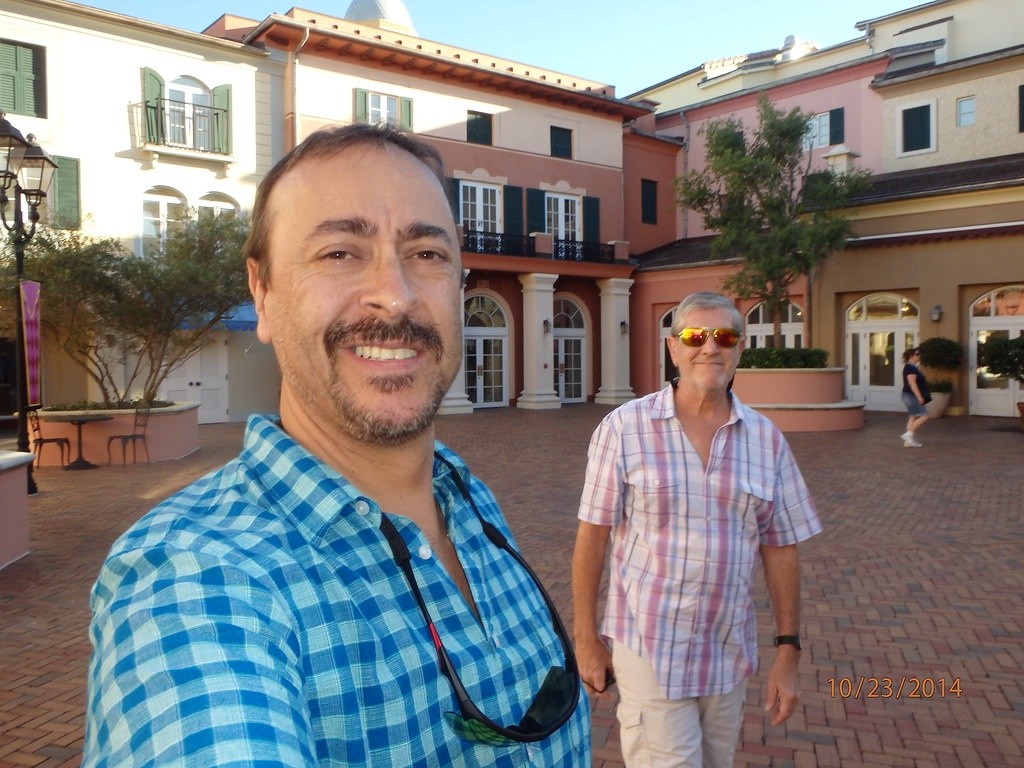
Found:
[774,635,802,649]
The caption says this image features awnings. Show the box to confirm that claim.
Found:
[145,290,258,332]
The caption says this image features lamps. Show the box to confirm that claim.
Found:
[620,320,629,334]
[929,304,944,321]
[543,318,551,333]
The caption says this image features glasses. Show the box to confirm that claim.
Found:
[401,523,580,747]
[914,353,920,356]
[672,327,744,347]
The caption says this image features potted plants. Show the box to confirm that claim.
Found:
[922,378,954,419]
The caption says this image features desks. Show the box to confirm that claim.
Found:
[42,414,114,471]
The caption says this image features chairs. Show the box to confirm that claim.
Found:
[106,402,152,469]
[28,410,72,472]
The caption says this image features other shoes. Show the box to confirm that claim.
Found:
[904,438,923,447]
[900,430,917,448]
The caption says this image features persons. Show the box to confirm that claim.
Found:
[719,331,729,344]
[691,332,706,345]
[571,290,822,768]
[81,123,592,768]
[901,349,933,447]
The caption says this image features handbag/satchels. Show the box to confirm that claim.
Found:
[920,391,933,405]
